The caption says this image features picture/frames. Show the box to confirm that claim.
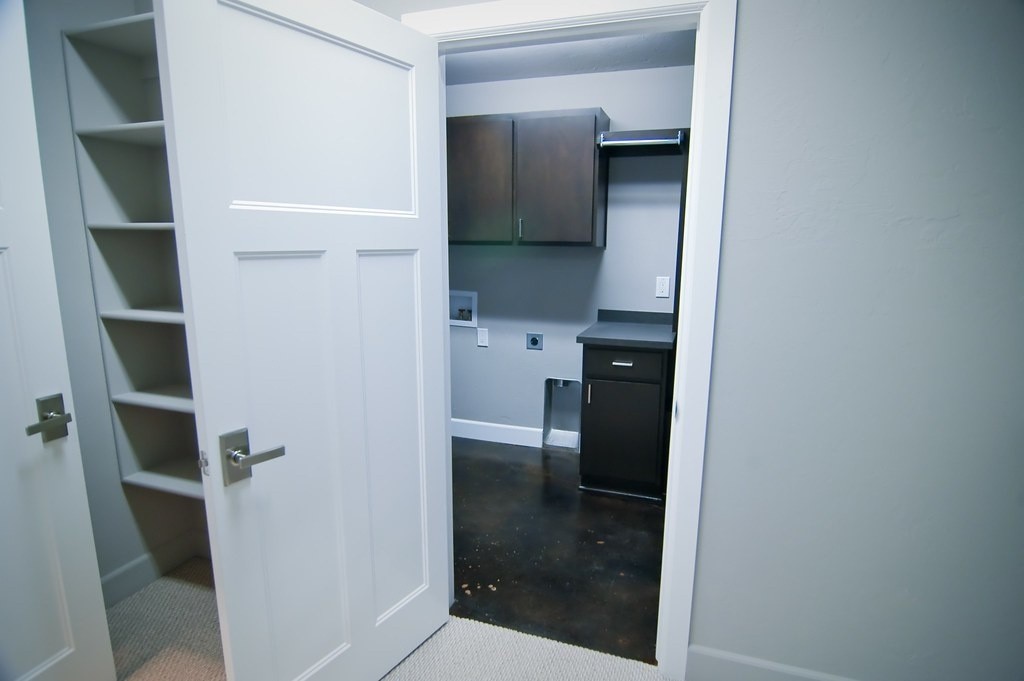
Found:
[449,289,478,329]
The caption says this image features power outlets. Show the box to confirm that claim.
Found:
[477,328,488,348]
[655,276,670,299]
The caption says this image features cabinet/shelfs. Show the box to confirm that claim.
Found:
[60,8,203,501]
[444,106,611,249]
[573,308,677,503]
